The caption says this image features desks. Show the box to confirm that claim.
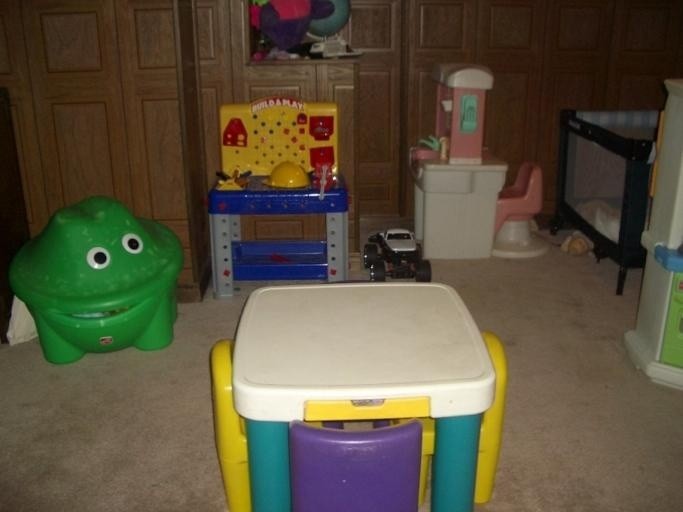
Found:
[232,282,496,511]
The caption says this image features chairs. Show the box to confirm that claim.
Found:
[209,334,325,511]
[295,422,421,511]
[493,164,551,260]
[420,334,508,506]
